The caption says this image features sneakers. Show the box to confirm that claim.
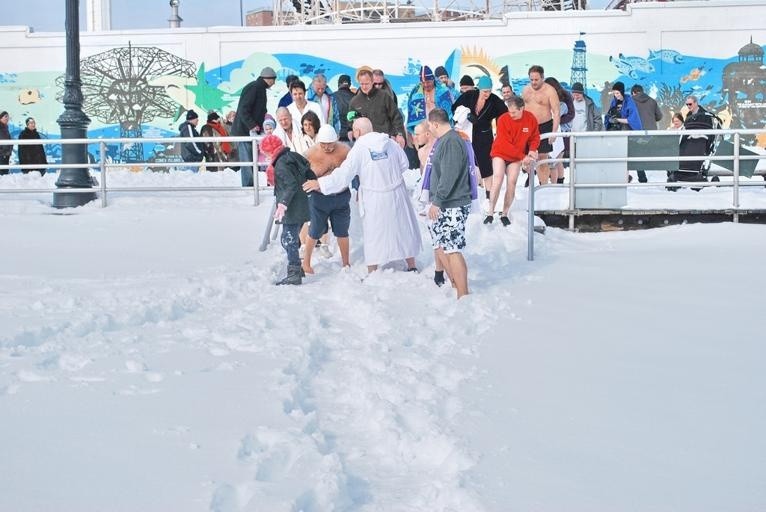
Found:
[484,216,493,224]
[320,245,332,258]
[501,217,510,225]
[435,272,445,287]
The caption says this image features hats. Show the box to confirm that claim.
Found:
[419,66,492,90]
[186,110,197,120]
[318,124,340,143]
[339,75,351,87]
[571,83,583,93]
[261,135,282,152]
[262,114,276,132]
[209,113,219,122]
[261,67,276,79]
[612,82,624,93]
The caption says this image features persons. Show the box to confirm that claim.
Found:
[258,135,322,286]
[412,121,477,288]
[18,118,49,177]
[0,111,13,176]
[299,123,354,275]
[425,106,475,303]
[175,62,724,227]
[300,116,424,275]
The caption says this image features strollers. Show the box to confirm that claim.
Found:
[663,113,725,191]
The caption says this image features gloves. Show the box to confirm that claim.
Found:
[274,202,287,221]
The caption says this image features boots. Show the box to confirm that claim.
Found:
[277,266,305,285]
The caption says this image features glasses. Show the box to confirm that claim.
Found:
[374,83,383,85]
[686,103,692,105]
